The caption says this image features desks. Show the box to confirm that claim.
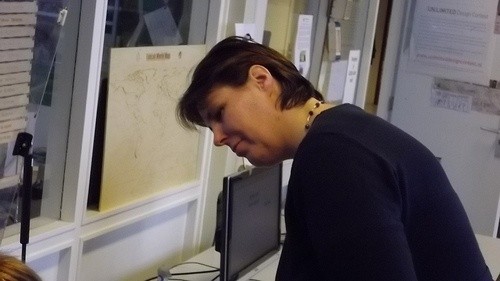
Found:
[154,216,500,281]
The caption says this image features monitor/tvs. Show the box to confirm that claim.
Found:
[215,161,282,281]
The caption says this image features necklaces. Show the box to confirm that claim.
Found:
[301,100,327,134]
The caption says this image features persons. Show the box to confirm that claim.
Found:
[177,36,495,281]
